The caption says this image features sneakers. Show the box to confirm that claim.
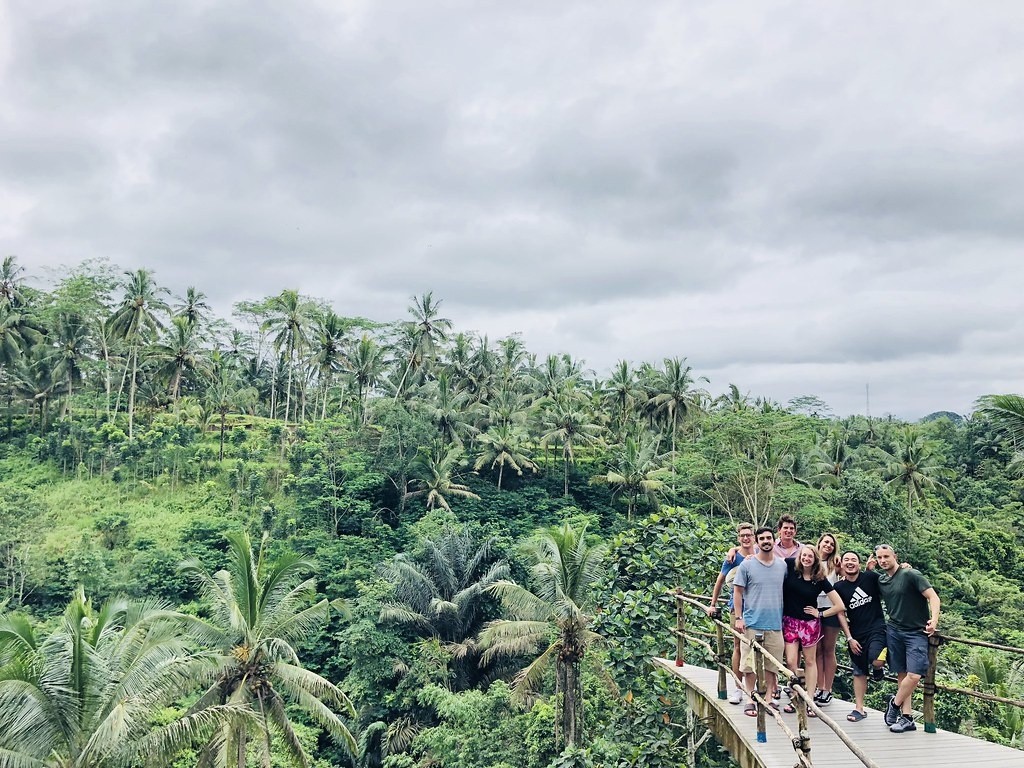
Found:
[814,688,834,705]
[884,695,902,726]
[890,716,916,733]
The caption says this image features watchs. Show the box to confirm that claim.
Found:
[734,616,742,621]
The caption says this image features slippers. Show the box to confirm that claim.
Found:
[783,688,795,697]
[873,664,884,681]
[744,704,757,717]
[764,703,780,716]
[847,710,867,721]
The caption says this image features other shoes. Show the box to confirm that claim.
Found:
[730,690,742,704]
[773,686,782,699]
[784,701,796,713]
[766,695,779,706]
[807,706,817,717]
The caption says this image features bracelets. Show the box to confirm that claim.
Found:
[818,611,824,618]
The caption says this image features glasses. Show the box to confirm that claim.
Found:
[739,534,754,537]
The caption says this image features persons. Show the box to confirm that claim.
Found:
[707,515,846,718]
[866,544,940,733]
[833,551,912,722]
